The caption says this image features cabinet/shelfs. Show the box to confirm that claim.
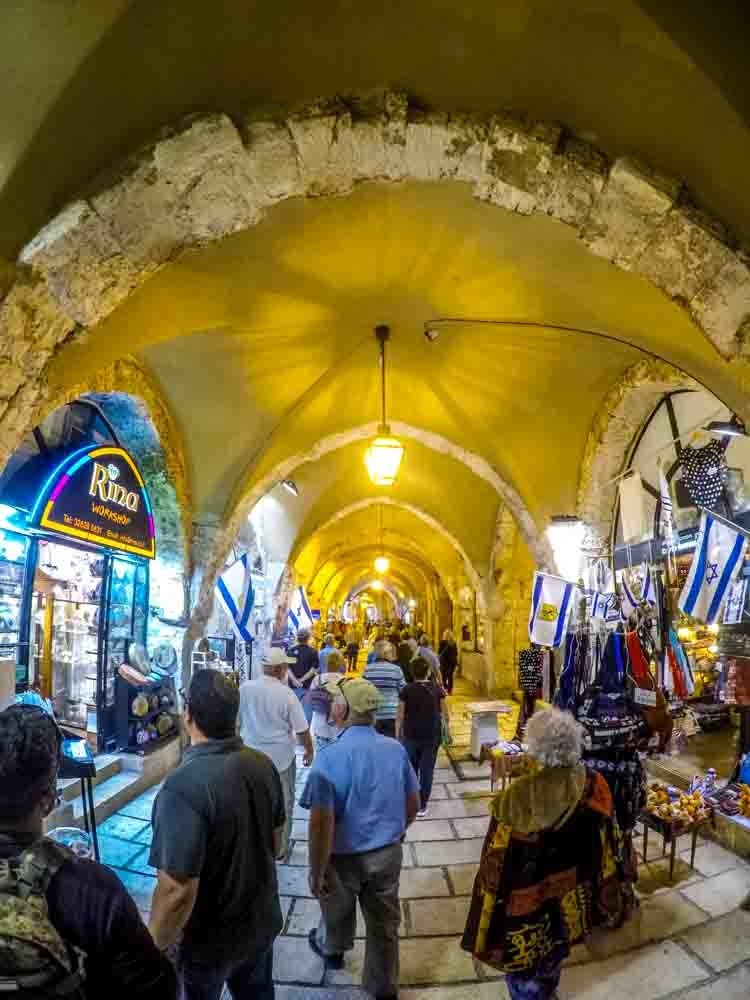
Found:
[115,677,178,752]
[0,527,145,743]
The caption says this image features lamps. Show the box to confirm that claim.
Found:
[281,479,297,497]
[706,413,746,437]
[359,324,405,485]
[547,514,581,526]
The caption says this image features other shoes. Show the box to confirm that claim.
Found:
[416,806,428,817]
[308,927,343,968]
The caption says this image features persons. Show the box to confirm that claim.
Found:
[297,675,422,1000]
[237,647,314,867]
[438,628,457,696]
[0,705,180,1000]
[271,613,448,755]
[395,658,453,817]
[460,712,628,1000]
[149,670,288,1000]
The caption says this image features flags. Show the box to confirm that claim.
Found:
[590,592,609,620]
[287,587,314,632]
[660,467,673,548]
[619,578,638,624]
[721,576,749,624]
[214,554,258,641]
[677,508,750,625]
[607,592,617,609]
[528,568,577,649]
[640,565,656,608]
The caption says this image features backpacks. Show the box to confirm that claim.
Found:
[0,837,91,1000]
[310,674,334,714]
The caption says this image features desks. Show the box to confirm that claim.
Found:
[464,700,512,760]
[637,804,709,881]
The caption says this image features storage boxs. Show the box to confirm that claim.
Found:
[480,743,524,777]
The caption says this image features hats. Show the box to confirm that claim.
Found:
[297,626,312,640]
[0,702,65,817]
[261,647,299,665]
[326,634,335,640]
[34,567,67,592]
[118,642,178,688]
[325,677,384,714]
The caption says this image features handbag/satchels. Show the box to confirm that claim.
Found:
[591,817,640,932]
[555,629,673,816]
[429,710,452,744]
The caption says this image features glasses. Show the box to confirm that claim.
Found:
[178,687,187,703]
[336,676,356,721]
